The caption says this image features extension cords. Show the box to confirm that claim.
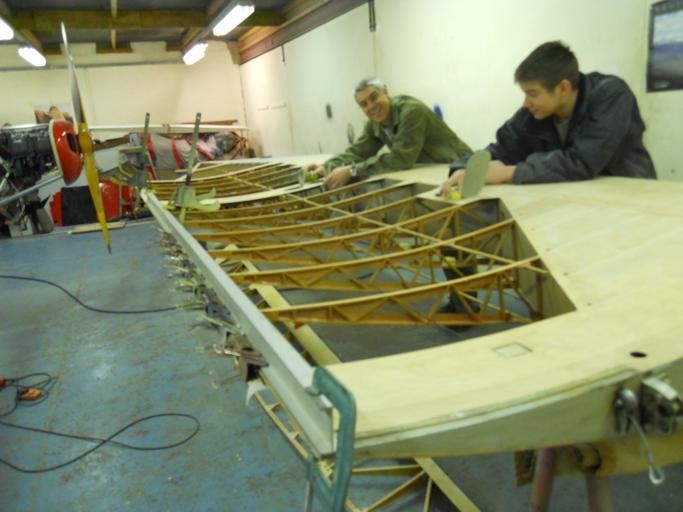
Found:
[17,387,42,400]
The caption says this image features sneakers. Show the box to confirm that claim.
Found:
[437,298,482,333]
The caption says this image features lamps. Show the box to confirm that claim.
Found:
[17,32,48,69]
[181,30,209,66]
[208,0,255,38]
[1,15,14,42]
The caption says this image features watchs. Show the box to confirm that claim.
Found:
[346,163,357,183]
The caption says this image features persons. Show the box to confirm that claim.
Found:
[436,41,657,202]
[301,77,498,334]
